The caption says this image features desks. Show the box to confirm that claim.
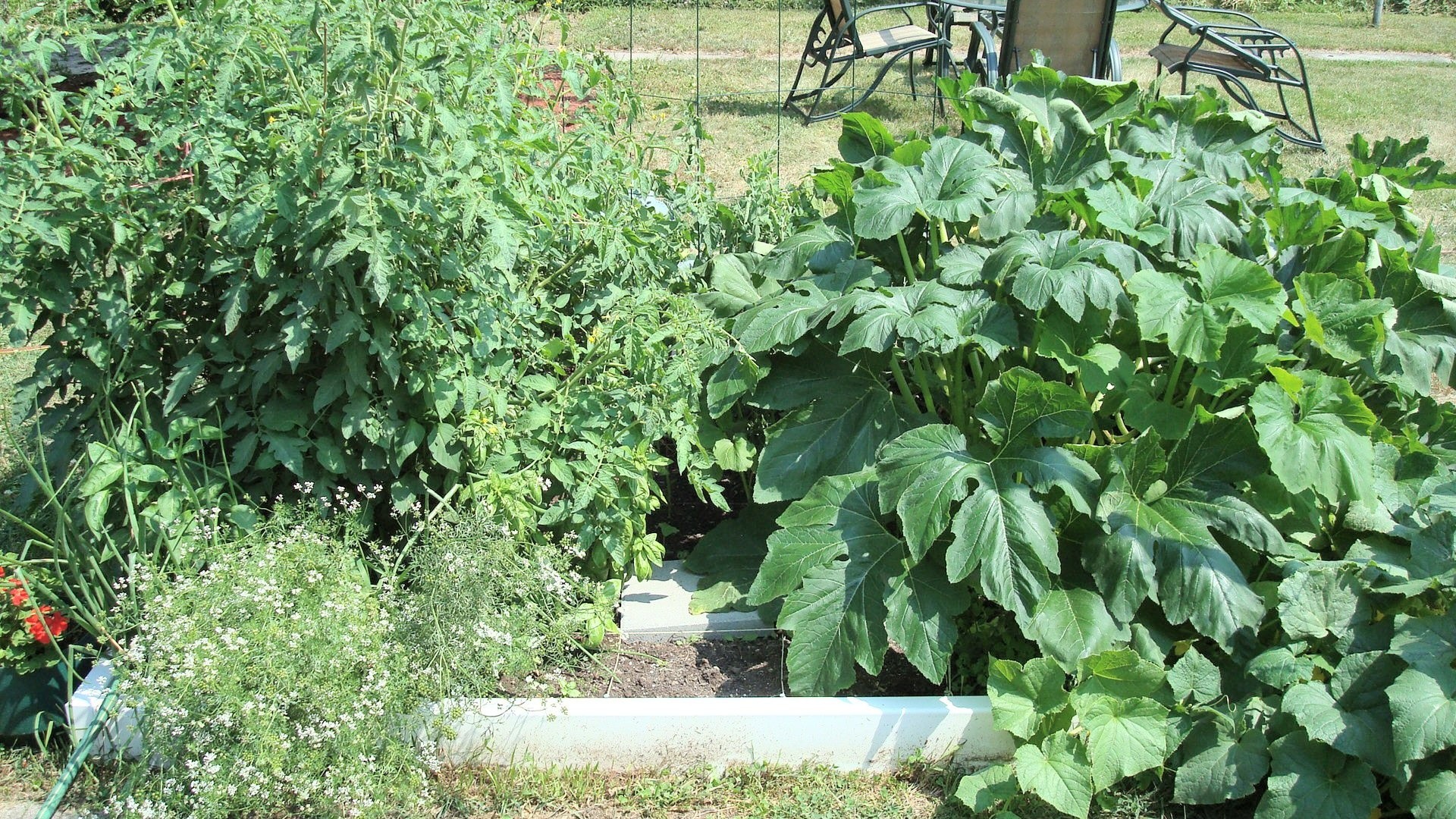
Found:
[923,0,1150,65]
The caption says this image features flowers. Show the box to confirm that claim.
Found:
[0,552,79,678]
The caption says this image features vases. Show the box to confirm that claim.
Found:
[0,632,95,737]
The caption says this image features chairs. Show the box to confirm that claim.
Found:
[961,0,1124,135]
[782,0,953,130]
[1148,0,1329,154]
